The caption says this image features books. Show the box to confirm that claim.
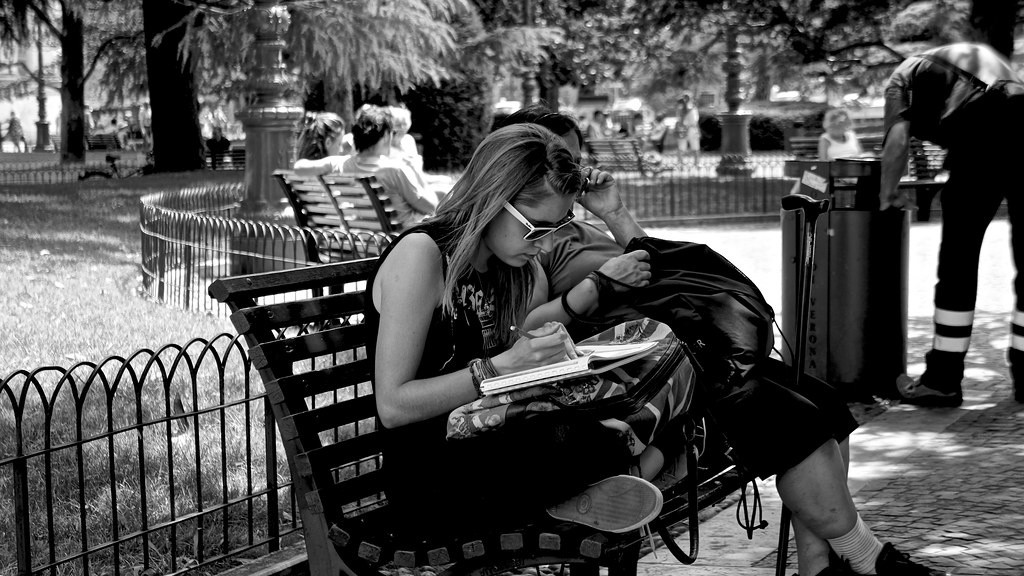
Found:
[480,343,658,399]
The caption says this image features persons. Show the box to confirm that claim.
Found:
[817,106,873,209]
[585,90,706,170]
[294,103,438,243]
[880,40,1024,409]
[6,110,24,155]
[205,127,230,170]
[344,104,454,202]
[93,119,119,136]
[364,122,694,542]
[500,104,946,576]
[295,111,345,159]
[84,105,95,148]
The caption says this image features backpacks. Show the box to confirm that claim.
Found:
[445,317,706,564]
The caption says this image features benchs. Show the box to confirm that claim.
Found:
[48,133,123,151]
[210,257,794,576]
[271,168,404,330]
[205,150,246,171]
[789,129,950,223]
[582,138,662,181]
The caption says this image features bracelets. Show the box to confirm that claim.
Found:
[587,272,599,302]
[468,357,499,398]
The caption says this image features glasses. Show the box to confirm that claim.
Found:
[504,202,576,242]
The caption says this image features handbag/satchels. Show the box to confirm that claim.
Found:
[562,236,775,431]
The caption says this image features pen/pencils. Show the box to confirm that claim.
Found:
[509,325,536,340]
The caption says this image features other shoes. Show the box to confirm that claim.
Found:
[876,542,947,576]
[546,475,664,560]
[792,555,861,576]
[896,373,962,408]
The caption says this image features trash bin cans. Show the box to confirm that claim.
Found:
[780,158,915,398]
